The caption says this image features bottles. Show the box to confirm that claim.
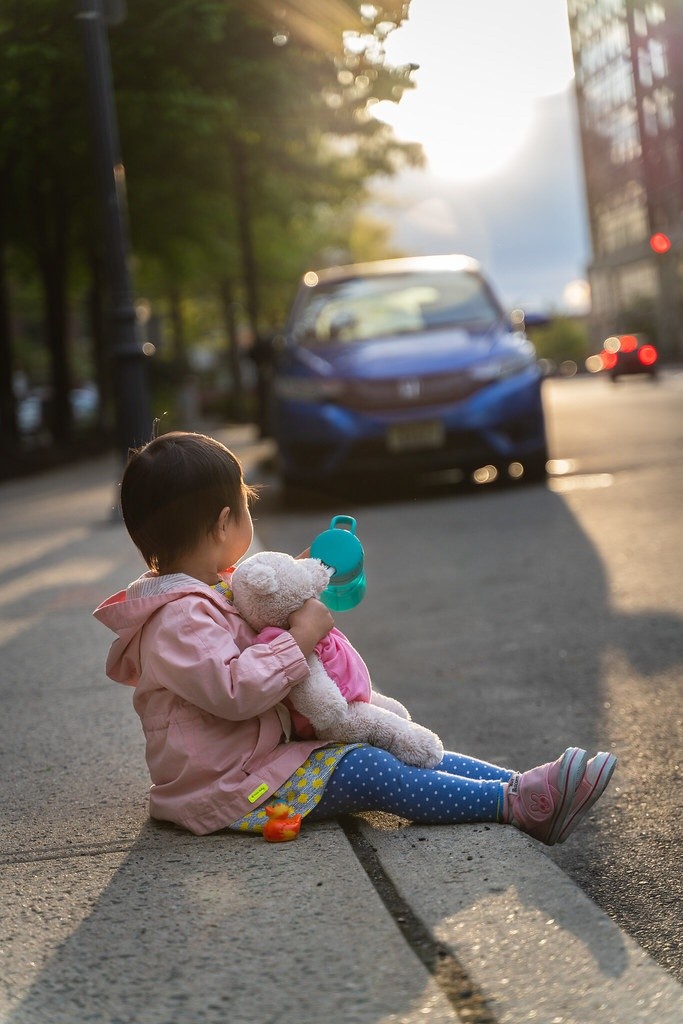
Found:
[310,515,365,614]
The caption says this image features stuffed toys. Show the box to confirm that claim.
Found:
[231,550,444,769]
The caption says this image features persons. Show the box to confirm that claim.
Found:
[93,431,619,846]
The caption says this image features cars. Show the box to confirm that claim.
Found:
[600,333,660,378]
[254,252,552,494]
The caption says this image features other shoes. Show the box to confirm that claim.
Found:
[507,747,588,846]
[557,752,618,844]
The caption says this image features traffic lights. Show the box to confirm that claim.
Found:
[650,233,674,253]
